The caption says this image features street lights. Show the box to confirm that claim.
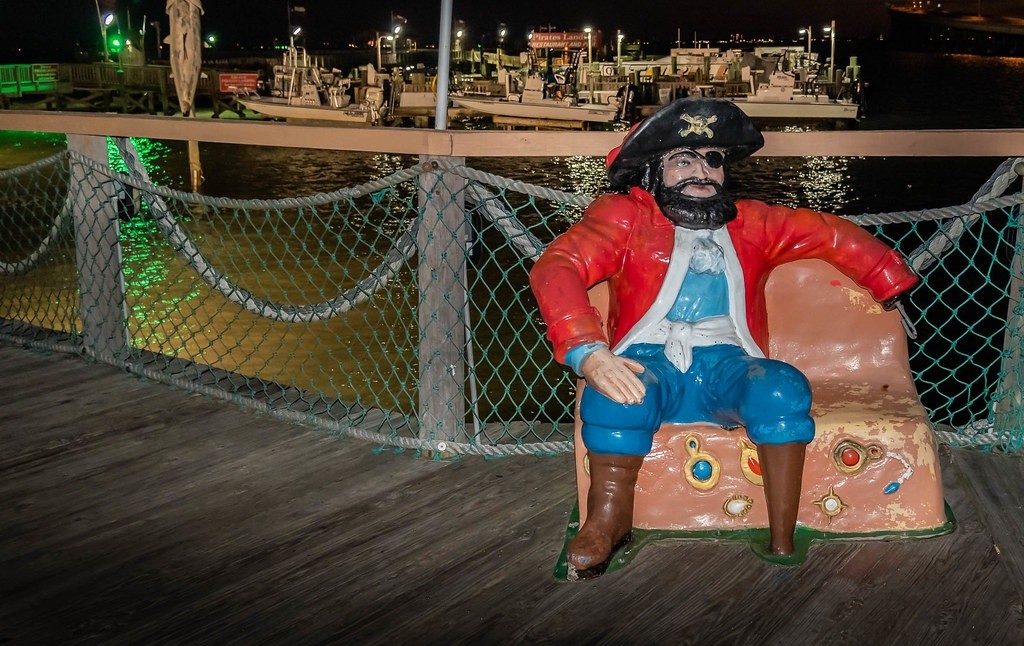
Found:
[291,27,303,48]
[822,20,836,82]
[617,29,624,67]
[377,36,394,73]
[800,26,811,72]
[583,27,592,70]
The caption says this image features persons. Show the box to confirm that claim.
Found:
[531,100,919,583]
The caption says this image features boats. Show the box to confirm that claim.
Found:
[225,22,861,133]
